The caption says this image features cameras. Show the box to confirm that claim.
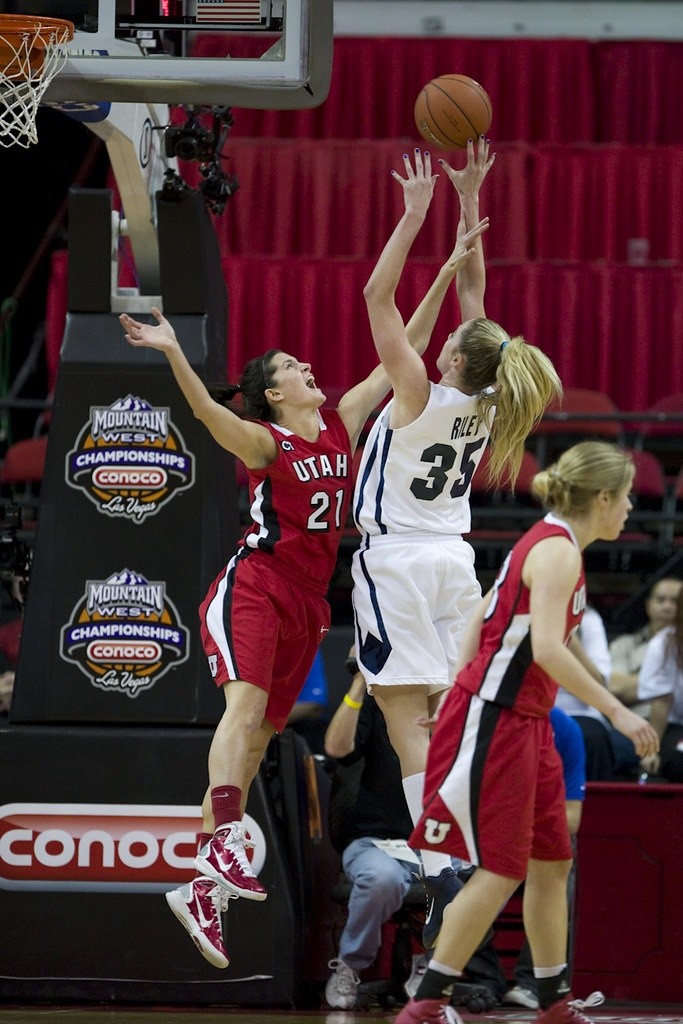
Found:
[165,124,210,162]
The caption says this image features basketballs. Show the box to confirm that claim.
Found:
[413,75,493,150]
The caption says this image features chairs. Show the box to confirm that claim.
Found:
[0,388,683,667]
[325,762,502,1012]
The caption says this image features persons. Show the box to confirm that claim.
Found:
[637,583,683,775]
[395,441,661,1024]
[609,580,683,780]
[553,608,614,782]
[464,704,586,1010]
[323,668,473,1008]
[283,650,329,724]
[351,134,565,951]
[119,209,490,969]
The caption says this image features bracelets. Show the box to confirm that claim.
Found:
[344,694,362,709]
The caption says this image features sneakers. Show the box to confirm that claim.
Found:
[502,981,541,1010]
[194,821,267,902]
[325,953,361,1008]
[404,952,432,998]
[165,874,239,968]
[395,995,464,1024]
[537,991,604,1024]
